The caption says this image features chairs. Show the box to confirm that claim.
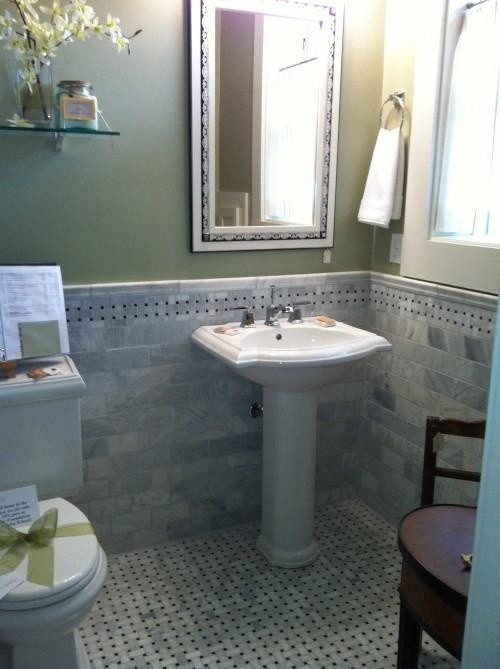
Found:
[420,416,485,507]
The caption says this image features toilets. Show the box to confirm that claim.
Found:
[0,354,109,669]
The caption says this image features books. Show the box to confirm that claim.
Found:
[0,263,71,365]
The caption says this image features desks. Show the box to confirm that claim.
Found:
[398,503,476,669]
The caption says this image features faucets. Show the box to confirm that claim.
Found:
[264,302,293,326]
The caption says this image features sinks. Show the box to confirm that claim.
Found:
[190,315,394,394]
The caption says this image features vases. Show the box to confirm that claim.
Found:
[13,50,54,128]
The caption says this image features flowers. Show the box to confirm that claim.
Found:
[0,1,145,119]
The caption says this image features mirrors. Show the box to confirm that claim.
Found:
[186,0,344,252]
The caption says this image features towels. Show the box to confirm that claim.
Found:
[356,126,411,231]
